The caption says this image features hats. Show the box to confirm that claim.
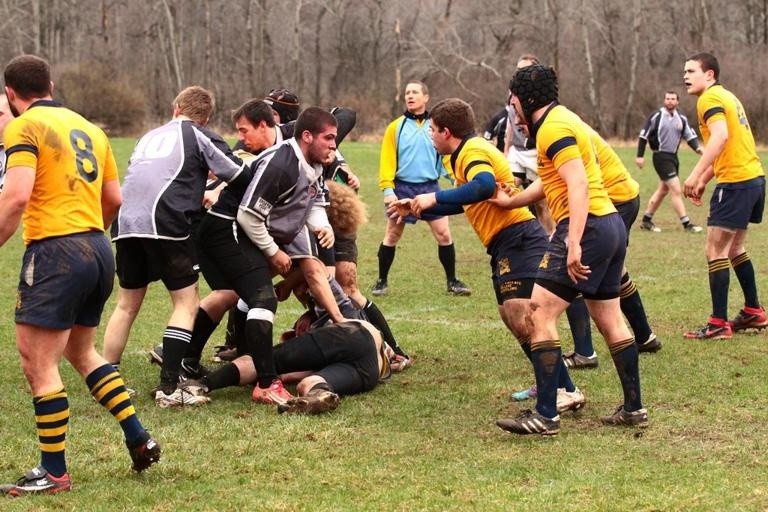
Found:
[509,62,560,117]
[261,87,300,124]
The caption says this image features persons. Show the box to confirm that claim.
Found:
[681,54,768,345]
[0,92,21,191]
[494,66,651,440]
[514,50,539,75]
[634,88,706,237]
[0,52,161,499]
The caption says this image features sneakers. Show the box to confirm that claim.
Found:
[638,220,662,233]
[511,385,538,402]
[277,388,340,416]
[682,313,733,341]
[601,404,651,430]
[125,431,163,475]
[728,305,768,334]
[684,221,703,233]
[632,332,664,354]
[372,279,389,296]
[555,386,587,414]
[495,406,561,436]
[561,350,599,370]
[146,342,239,410]
[447,278,472,297]
[0,463,71,499]
[384,342,411,374]
[251,378,297,406]
